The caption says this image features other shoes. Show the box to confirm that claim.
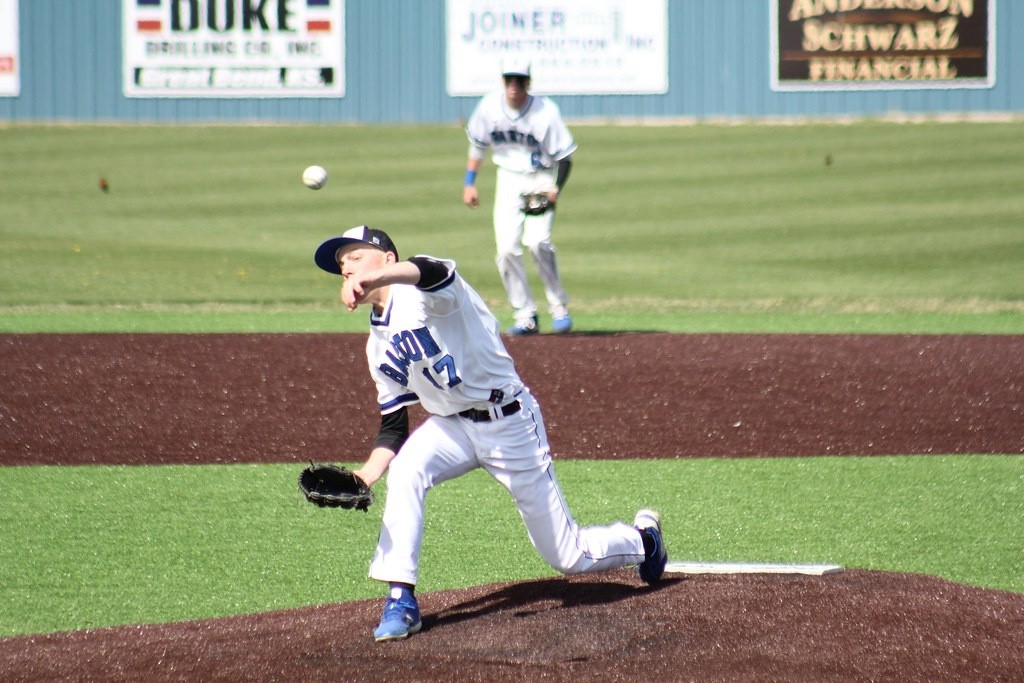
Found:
[549,304,572,334]
[508,313,539,335]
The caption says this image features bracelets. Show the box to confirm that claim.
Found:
[465,171,477,185]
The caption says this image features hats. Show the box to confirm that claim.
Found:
[313,224,399,275]
[501,55,530,76]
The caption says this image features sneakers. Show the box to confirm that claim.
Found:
[373,595,422,642]
[634,509,668,585]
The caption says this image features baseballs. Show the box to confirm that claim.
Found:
[303,166,328,190]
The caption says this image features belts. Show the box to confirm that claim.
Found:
[458,400,521,422]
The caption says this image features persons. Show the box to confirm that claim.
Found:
[463,58,577,336]
[300,225,668,643]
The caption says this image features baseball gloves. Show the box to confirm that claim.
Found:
[298,462,375,509]
[518,190,556,215]
